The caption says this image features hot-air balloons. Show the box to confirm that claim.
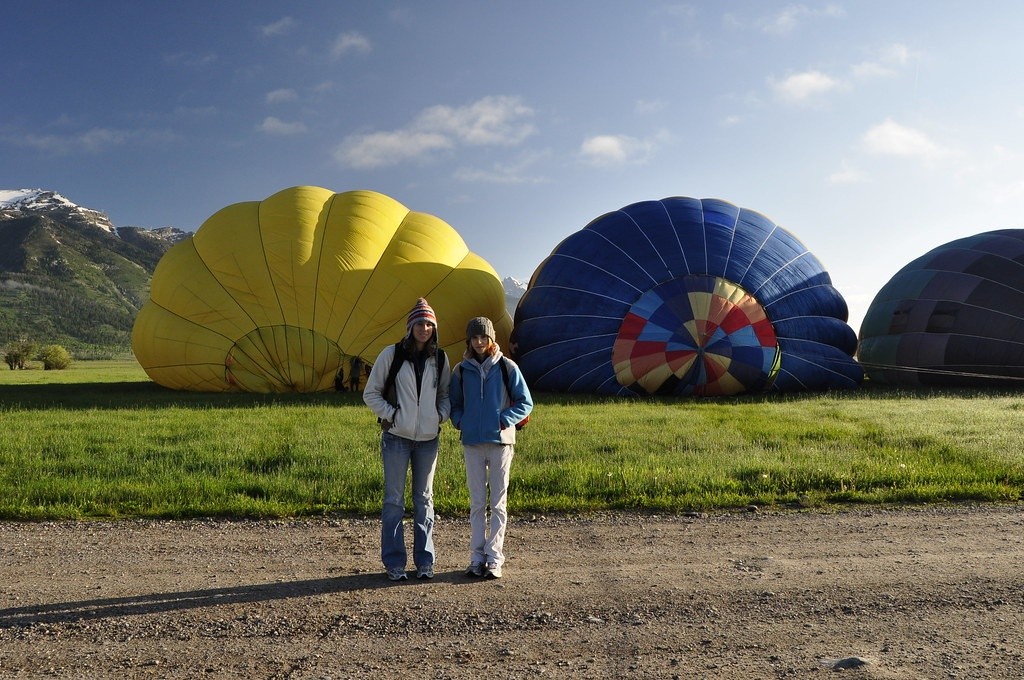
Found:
[857,227,1023,393]
[130,183,515,398]
[509,196,871,403]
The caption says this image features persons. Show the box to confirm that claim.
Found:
[364,298,450,582]
[335,356,371,392]
[449,317,534,578]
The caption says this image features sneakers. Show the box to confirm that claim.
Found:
[417,564,434,579]
[386,568,409,582]
[484,560,502,578]
[465,561,483,577]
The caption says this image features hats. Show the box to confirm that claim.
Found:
[466,317,496,343]
[405,297,438,388]
